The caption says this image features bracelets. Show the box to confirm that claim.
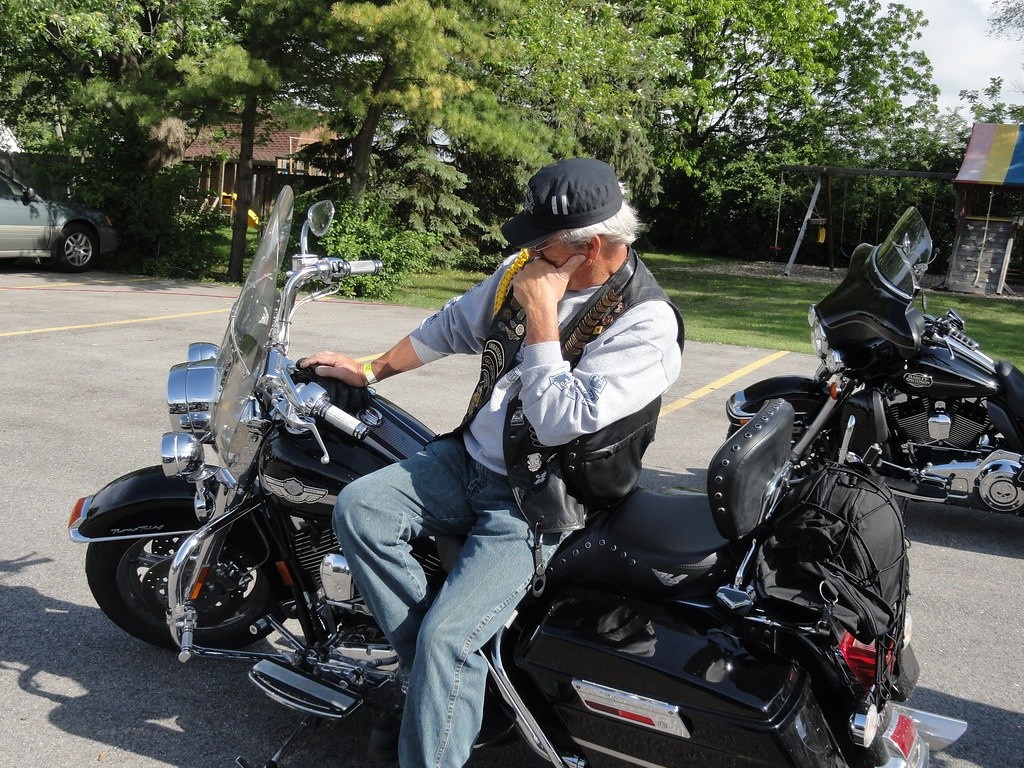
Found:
[363,362,378,385]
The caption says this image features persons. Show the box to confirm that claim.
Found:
[299,158,685,768]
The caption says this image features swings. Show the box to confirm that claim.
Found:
[785,171,828,244]
[876,177,903,246]
[915,180,939,232]
[839,174,868,258]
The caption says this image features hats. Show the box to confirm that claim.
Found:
[501,157,623,249]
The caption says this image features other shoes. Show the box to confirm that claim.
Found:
[367,695,404,763]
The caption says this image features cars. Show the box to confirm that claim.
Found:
[0,171,120,275]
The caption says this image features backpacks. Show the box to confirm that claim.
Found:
[771,456,913,642]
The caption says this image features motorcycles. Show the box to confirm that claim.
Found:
[723,204,1023,514]
[65,182,970,768]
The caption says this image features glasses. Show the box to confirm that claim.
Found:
[532,239,563,254]
